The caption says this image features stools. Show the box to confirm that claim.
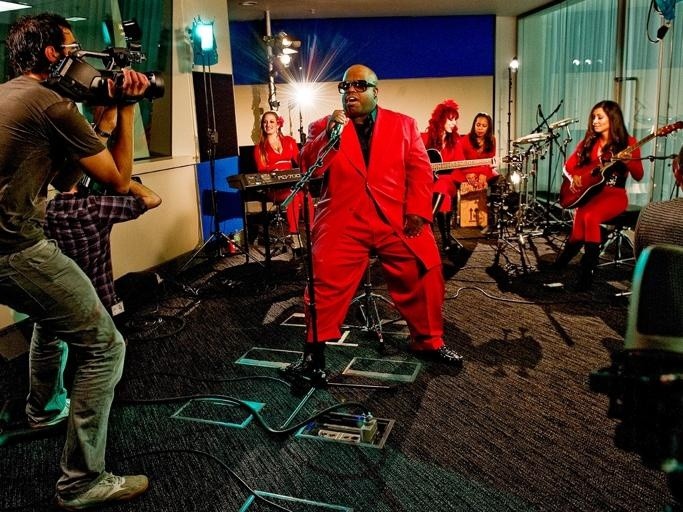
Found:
[593,206,641,267]
[343,246,412,353]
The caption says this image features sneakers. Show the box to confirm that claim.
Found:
[55,473,148,510]
[28,399,70,429]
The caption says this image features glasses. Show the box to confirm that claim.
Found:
[60,41,82,50]
[338,80,375,94]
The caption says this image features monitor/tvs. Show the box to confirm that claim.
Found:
[191,71,238,163]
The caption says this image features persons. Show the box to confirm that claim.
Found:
[280,65,463,380]
[463,112,501,229]
[0,12,148,510]
[550,100,645,292]
[11,156,162,382]
[254,113,317,257]
[419,99,465,253]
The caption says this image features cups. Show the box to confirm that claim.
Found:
[230,239,236,254]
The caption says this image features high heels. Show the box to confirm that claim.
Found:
[290,246,305,257]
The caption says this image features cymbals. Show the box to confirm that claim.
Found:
[549,117,576,129]
[515,133,554,142]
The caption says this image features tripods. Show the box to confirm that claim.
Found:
[278,188,390,430]
[174,130,266,275]
[486,116,582,284]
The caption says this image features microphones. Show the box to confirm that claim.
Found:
[331,124,341,137]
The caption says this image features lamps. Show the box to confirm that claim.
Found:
[179,14,243,275]
[505,55,519,171]
[264,9,301,111]
[100,17,142,47]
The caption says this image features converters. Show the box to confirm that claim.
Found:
[358,419,378,442]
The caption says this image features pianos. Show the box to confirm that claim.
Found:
[226,169,324,189]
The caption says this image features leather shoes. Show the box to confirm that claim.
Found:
[416,345,463,363]
[277,352,324,377]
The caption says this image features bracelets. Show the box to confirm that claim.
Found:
[89,121,111,139]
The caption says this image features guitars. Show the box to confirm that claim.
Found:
[559,121,682,208]
[426,148,498,180]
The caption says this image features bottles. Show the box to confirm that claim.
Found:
[233,229,241,250]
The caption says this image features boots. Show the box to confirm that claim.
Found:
[564,242,600,293]
[431,192,444,216]
[435,211,460,253]
[540,239,585,272]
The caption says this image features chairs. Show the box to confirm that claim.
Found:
[239,143,302,259]
[604,242,683,471]
